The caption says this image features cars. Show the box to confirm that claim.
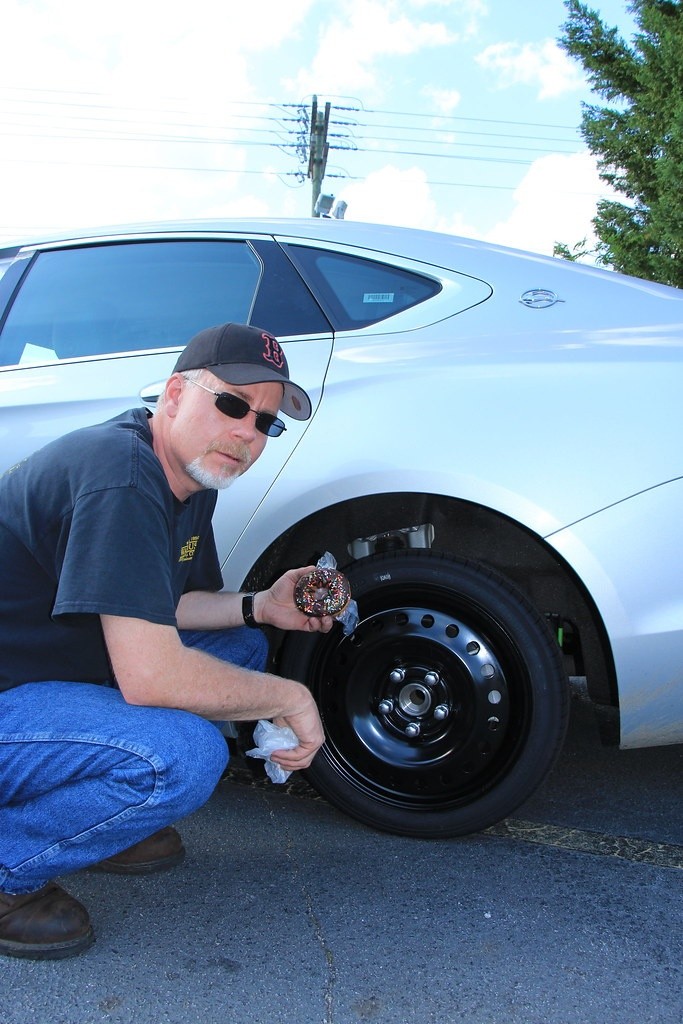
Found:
[1,220,682,843]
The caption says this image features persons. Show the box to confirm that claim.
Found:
[0,324,334,961]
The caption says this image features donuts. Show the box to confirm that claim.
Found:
[295,567,351,617]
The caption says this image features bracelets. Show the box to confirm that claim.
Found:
[241,591,260,629]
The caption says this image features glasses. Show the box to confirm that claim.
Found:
[184,377,287,437]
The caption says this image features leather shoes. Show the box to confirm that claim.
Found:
[0,880,96,958]
[85,824,185,872]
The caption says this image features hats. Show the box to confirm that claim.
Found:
[171,322,312,421]
